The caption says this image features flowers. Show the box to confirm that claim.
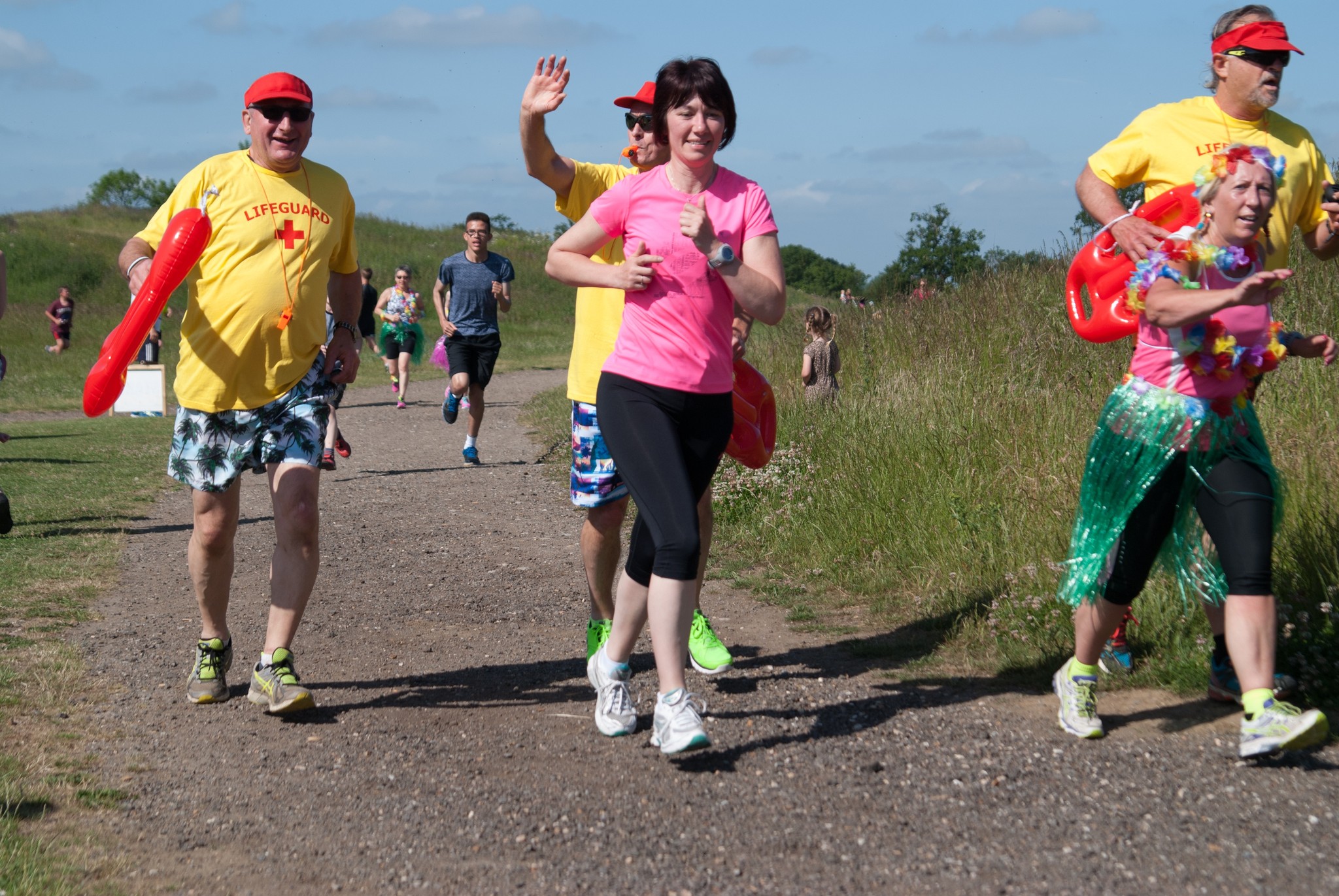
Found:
[1192,143,1286,188]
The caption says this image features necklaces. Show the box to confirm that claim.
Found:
[668,162,716,202]
[395,284,416,323]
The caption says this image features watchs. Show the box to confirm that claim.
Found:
[708,243,735,270]
[332,321,358,343]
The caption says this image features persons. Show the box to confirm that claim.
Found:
[1052,4,1339,758]
[0,251,14,534]
[545,56,788,754]
[800,306,841,406]
[520,54,733,675]
[118,71,515,715]
[43,286,75,354]
[840,277,936,318]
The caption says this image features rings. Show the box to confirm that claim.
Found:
[640,276,644,285]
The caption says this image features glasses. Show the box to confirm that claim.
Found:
[466,228,489,237]
[624,113,655,132]
[395,275,410,280]
[247,104,312,123]
[1221,50,1291,67]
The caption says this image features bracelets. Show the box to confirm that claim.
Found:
[380,310,387,320]
[126,256,149,280]
[1325,218,1336,242]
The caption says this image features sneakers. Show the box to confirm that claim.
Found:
[320,453,338,471]
[392,378,400,393]
[1096,603,1139,678]
[186,636,234,701]
[1206,652,1293,706]
[462,445,481,466]
[689,610,734,675]
[1052,655,1105,737]
[587,648,638,735]
[1238,687,1327,757]
[459,396,470,409]
[444,380,452,398]
[396,396,407,409]
[246,647,314,713]
[585,618,613,661]
[442,388,464,424]
[651,687,709,752]
[334,428,351,458]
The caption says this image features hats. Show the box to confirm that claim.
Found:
[1211,20,1305,56]
[246,72,312,103]
[613,80,656,108]
